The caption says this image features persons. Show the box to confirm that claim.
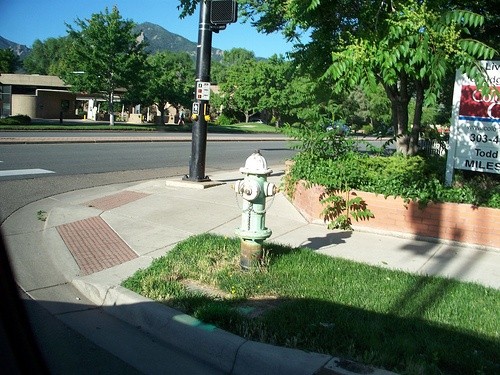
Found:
[177,108,186,125]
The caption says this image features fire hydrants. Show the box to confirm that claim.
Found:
[230,150,277,273]
